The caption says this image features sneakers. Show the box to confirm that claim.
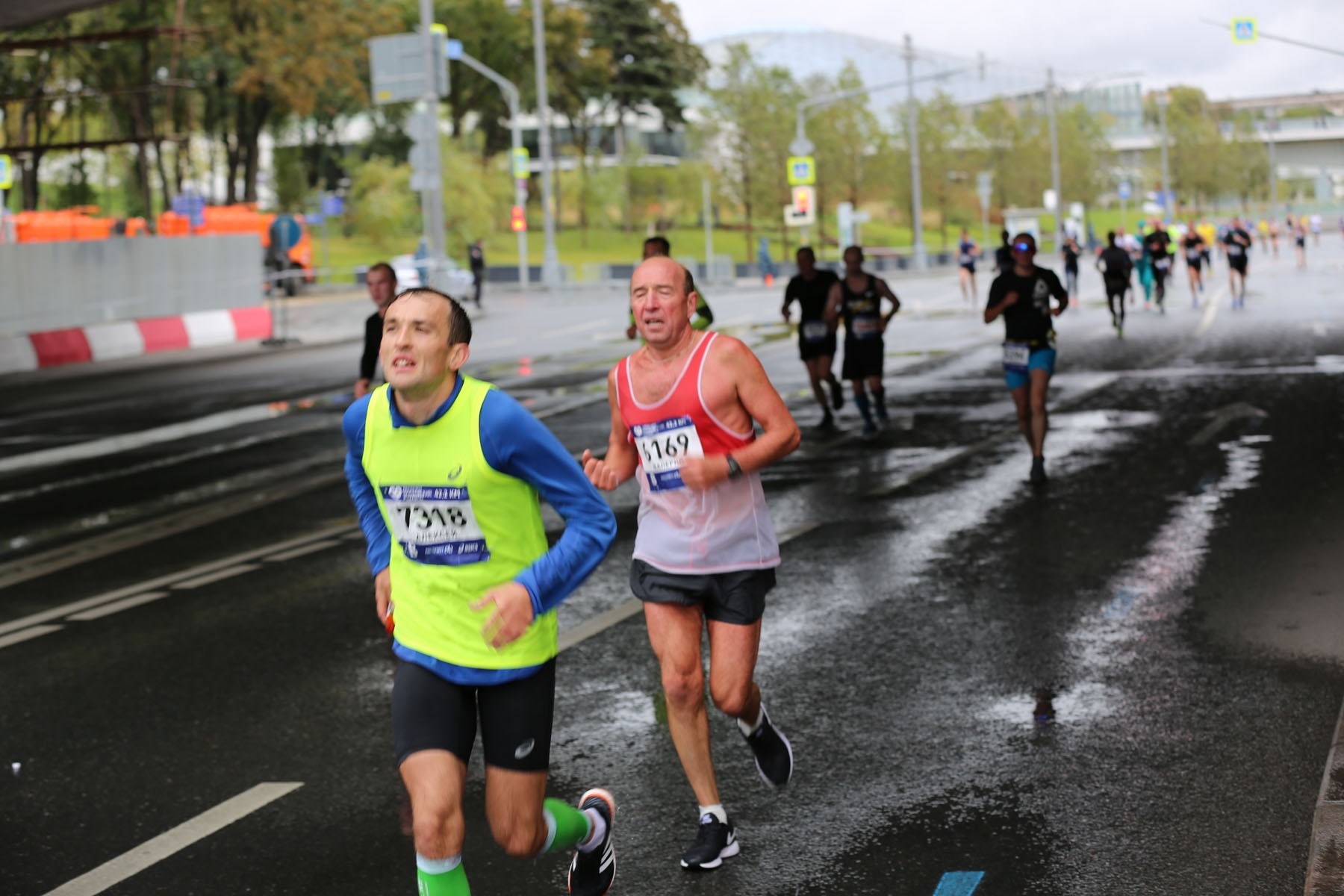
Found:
[681,812,739,870]
[567,788,616,896]
[735,703,792,786]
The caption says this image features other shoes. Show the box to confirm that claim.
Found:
[862,420,876,435]
[1192,298,1199,306]
[1198,284,1204,292]
[1118,328,1124,338]
[831,383,844,409]
[1113,313,1118,326]
[821,411,834,426]
[1031,456,1044,480]
[877,404,887,419]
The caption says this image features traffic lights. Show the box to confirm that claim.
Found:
[512,206,528,232]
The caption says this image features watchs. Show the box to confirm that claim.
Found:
[723,453,744,479]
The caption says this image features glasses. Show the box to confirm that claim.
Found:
[1012,245,1035,254]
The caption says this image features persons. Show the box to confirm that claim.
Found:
[576,253,794,867]
[342,285,617,896]
[351,211,1324,484]
